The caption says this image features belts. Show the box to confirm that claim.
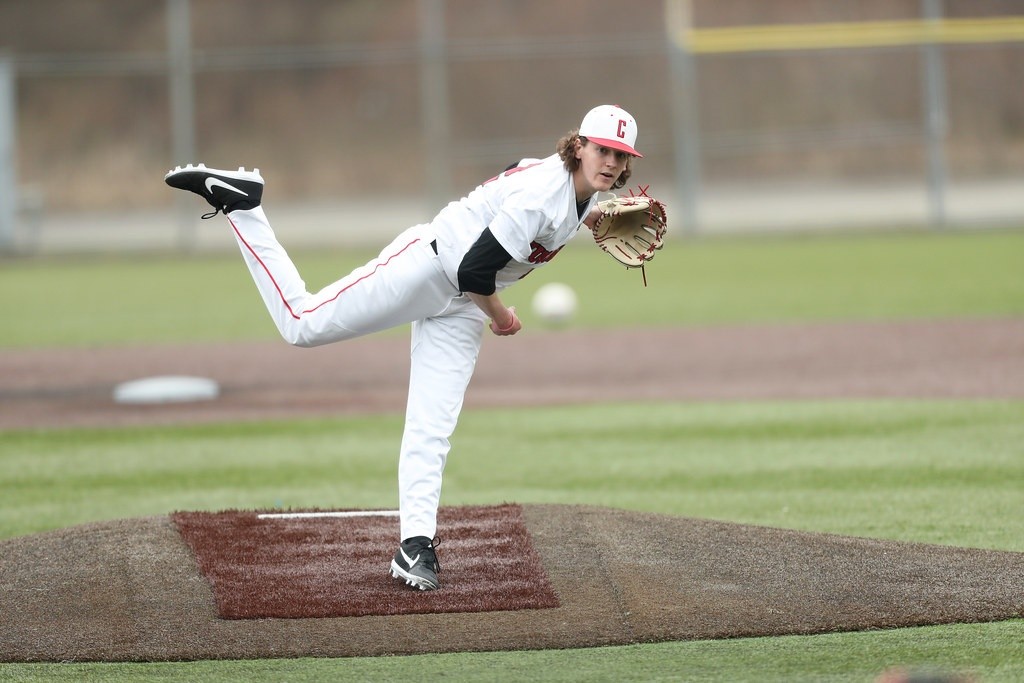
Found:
[430,239,438,255]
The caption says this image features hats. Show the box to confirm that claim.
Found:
[578,104,644,158]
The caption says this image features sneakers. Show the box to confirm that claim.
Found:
[389,535,441,591]
[164,164,265,220]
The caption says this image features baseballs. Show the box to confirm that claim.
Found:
[533,282,579,325]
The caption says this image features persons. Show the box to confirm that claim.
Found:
[164,104,642,589]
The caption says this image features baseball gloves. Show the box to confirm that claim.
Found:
[591,190,671,271]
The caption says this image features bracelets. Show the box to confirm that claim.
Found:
[491,309,515,333]
